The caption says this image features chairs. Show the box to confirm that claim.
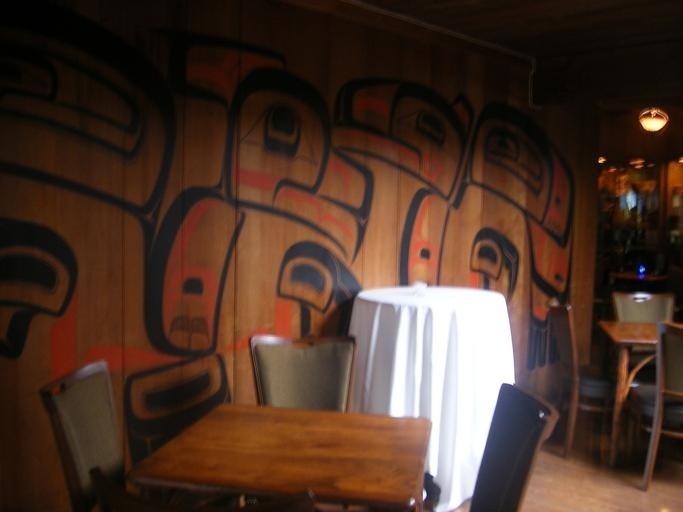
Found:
[39,358,200,512]
[549,292,683,492]
[467,380,561,511]
[248,333,443,512]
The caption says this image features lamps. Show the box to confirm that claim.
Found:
[638,107,671,134]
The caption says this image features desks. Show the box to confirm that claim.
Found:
[346,284,516,512]
[122,401,433,512]
